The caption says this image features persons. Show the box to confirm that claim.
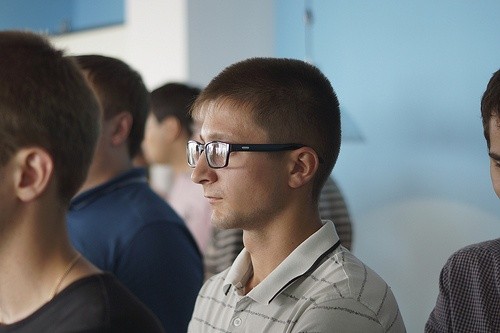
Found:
[188,57,407,333]
[424,69,500,333]
[0,28,353,333]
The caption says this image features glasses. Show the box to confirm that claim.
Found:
[186,140,308,169]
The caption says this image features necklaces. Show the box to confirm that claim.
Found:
[53,253,81,297]
